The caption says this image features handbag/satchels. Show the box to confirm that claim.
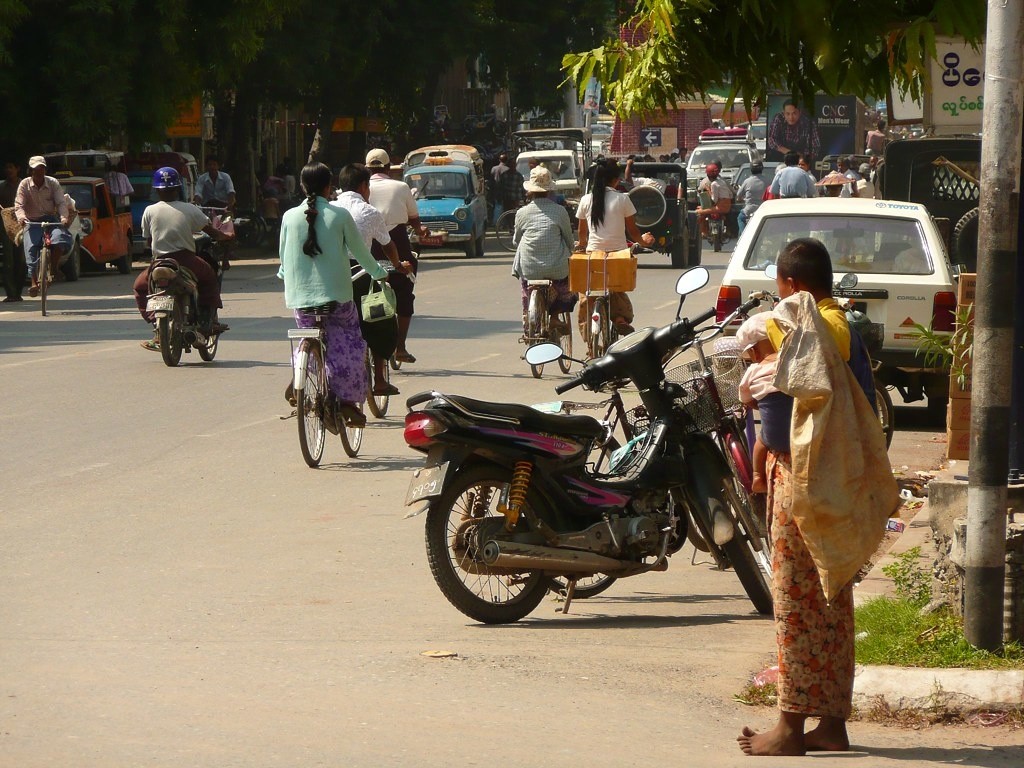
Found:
[361,278,396,322]
[212,206,235,238]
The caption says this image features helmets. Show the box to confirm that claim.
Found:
[858,163,871,174]
[152,167,182,187]
[706,165,719,177]
[711,160,722,169]
[750,160,763,172]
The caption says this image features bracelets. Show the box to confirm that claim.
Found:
[395,261,401,270]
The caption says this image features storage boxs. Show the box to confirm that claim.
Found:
[947,273,978,459]
[569,248,637,292]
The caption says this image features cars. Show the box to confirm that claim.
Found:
[716,197,955,415]
[732,162,786,213]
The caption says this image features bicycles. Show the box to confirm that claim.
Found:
[23,218,66,316]
[193,201,266,246]
[278,237,654,469]
[548,296,765,597]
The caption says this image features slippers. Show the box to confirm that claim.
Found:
[206,325,225,335]
[617,322,634,336]
[373,384,400,396]
[141,341,161,351]
[397,354,415,363]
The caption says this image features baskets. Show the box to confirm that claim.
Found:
[622,347,744,444]
[851,320,885,352]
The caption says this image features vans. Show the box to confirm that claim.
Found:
[686,129,763,207]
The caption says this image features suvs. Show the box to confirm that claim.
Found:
[615,162,702,269]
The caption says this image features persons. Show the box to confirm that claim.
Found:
[104,162,134,195]
[628,155,656,161]
[278,163,390,426]
[490,153,523,236]
[13,155,69,297]
[735,238,878,755]
[329,163,414,395]
[574,158,656,354]
[865,119,886,155]
[0,160,27,302]
[660,147,689,164]
[366,149,431,362]
[695,160,733,238]
[767,100,821,158]
[736,160,769,232]
[872,111,881,123]
[624,159,665,193]
[262,158,297,218]
[762,152,878,253]
[193,154,235,271]
[510,165,574,339]
[133,167,236,351]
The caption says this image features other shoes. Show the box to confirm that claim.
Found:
[29,286,37,297]
[285,382,294,401]
[222,261,230,270]
[342,404,366,424]
[552,321,569,335]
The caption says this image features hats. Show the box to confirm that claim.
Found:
[523,167,557,192]
[735,310,776,353]
[366,148,389,167]
[29,156,47,167]
[814,170,855,186]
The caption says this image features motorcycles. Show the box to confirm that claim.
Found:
[402,267,773,622]
[53,176,133,280]
[145,234,234,365]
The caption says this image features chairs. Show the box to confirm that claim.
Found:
[875,241,913,271]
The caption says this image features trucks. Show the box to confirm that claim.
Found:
[510,128,592,201]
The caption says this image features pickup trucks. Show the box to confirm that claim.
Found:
[404,166,488,259]
